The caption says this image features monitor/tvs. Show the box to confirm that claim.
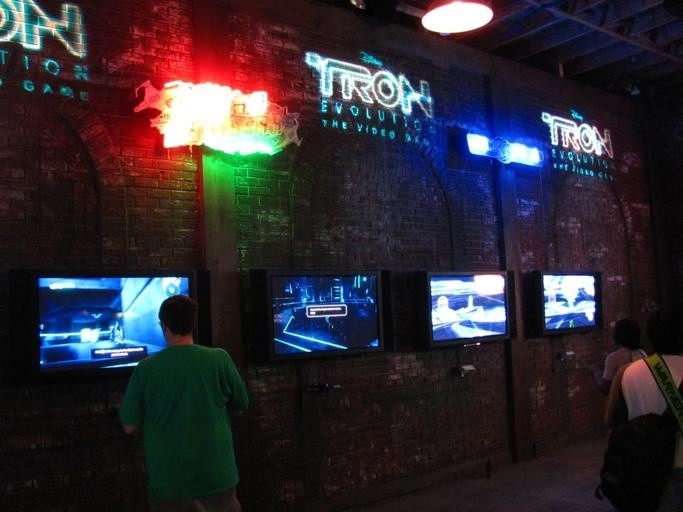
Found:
[531,269,604,338]
[25,270,198,382]
[419,269,512,349]
[255,268,385,363]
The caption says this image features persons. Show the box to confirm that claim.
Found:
[605,310,683,512]
[118,295,251,512]
[598,318,649,395]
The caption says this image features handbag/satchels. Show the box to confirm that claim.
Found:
[594,412,677,511]
